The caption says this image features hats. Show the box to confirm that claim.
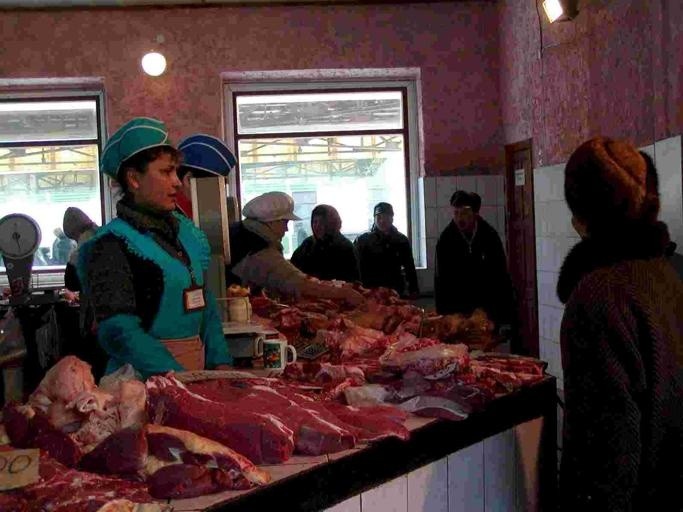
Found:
[176,135,237,181]
[449,190,481,214]
[564,137,662,233]
[373,202,394,216]
[242,191,301,223]
[62,207,92,238]
[99,117,177,179]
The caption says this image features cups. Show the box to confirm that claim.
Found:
[262,339,297,373]
[254,332,280,362]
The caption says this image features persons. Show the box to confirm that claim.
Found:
[62,204,104,255]
[349,202,422,300]
[555,132,683,510]
[290,205,361,287]
[222,191,365,306]
[432,190,520,340]
[169,131,226,223]
[74,116,233,379]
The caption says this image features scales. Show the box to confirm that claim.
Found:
[0,213,65,308]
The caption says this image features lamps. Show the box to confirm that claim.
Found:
[540,1,576,25]
